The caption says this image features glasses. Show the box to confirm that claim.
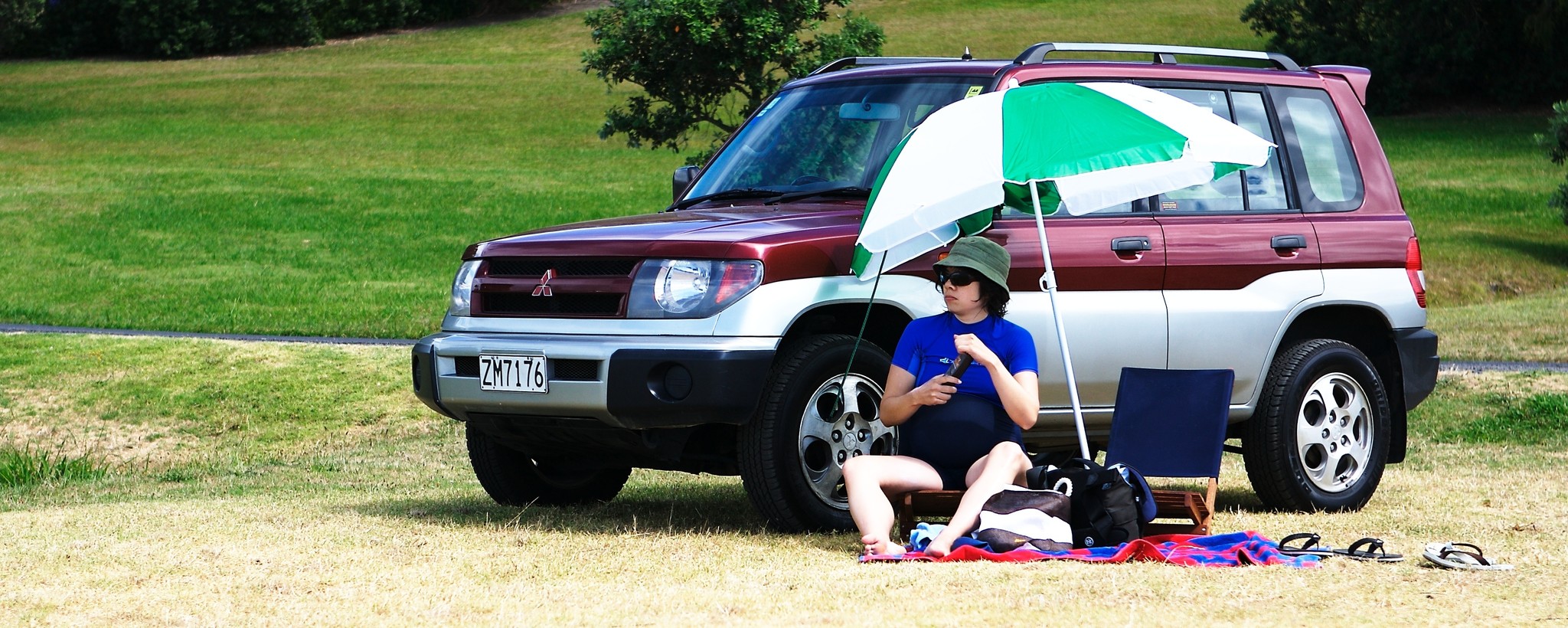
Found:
[939,270,982,286]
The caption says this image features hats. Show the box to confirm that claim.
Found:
[932,236,1010,302]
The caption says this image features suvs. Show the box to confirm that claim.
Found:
[414,40,1444,537]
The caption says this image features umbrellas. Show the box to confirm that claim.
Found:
[846,78,1278,474]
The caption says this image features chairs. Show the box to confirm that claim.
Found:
[1105,367,1235,535]
[899,490,966,543]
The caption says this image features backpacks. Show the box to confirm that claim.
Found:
[1025,460,1157,549]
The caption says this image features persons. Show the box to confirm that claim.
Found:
[839,234,1040,558]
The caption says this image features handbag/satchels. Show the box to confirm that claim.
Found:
[978,477,1075,556]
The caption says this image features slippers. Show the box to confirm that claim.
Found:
[1424,541,1495,565]
[1276,532,1334,556]
[1332,538,1405,561]
[1423,550,1514,571]
